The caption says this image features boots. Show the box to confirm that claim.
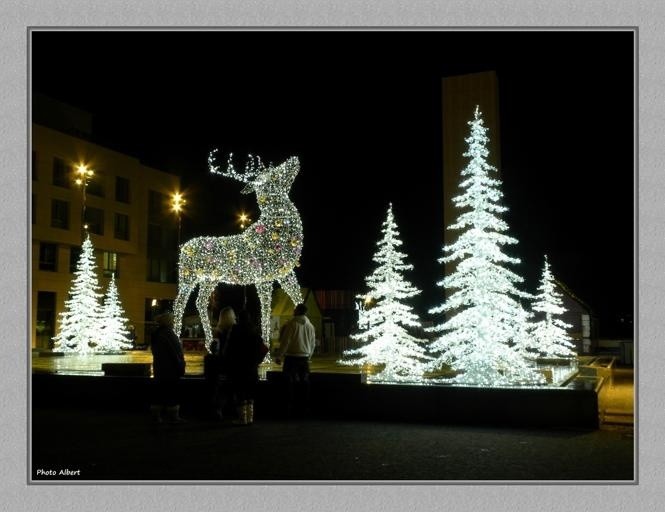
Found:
[245,400,255,424]
[231,400,248,426]
[167,404,181,423]
[150,405,164,429]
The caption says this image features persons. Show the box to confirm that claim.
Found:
[222,311,268,424]
[150,312,189,424]
[204,305,238,421]
[275,303,318,372]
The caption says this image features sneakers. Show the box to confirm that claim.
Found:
[215,409,226,424]
